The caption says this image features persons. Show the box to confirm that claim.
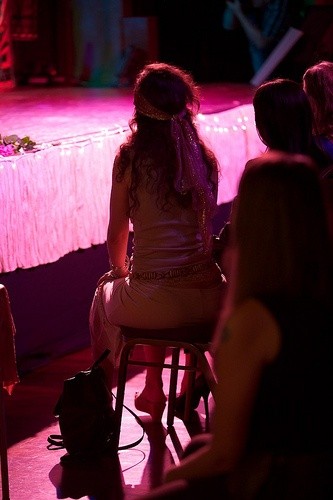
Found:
[224,0,306,82]
[168,77,333,420]
[90,60,227,423]
[133,150,333,500]
[303,60,333,166]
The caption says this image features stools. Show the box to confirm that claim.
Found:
[108,326,215,456]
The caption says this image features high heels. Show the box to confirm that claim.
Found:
[174,372,211,420]
[135,390,167,424]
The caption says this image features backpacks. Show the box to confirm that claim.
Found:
[47,349,146,478]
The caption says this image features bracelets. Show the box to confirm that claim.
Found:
[108,254,130,278]
